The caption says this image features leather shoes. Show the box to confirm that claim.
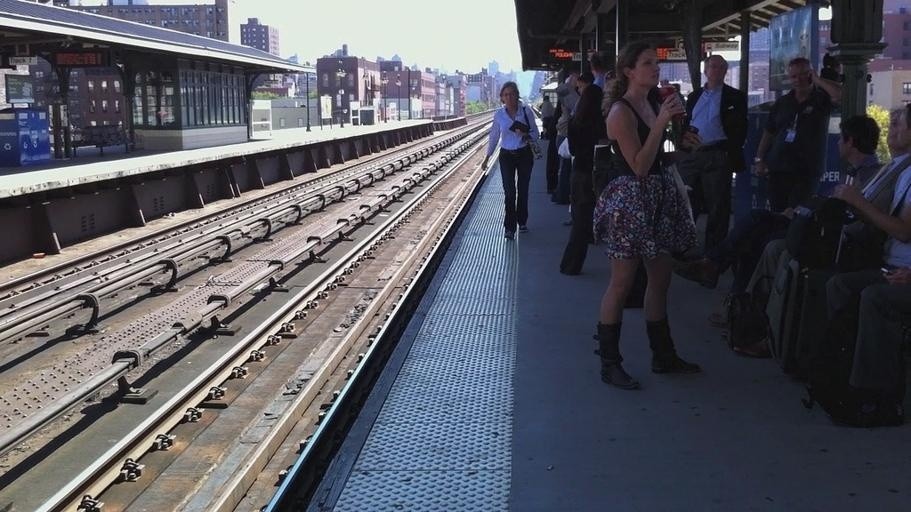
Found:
[708,292,748,330]
[672,258,719,290]
[732,334,771,359]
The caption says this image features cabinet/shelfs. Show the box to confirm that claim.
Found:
[0,106,51,167]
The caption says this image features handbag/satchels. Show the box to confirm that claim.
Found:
[801,198,845,240]
[527,136,543,159]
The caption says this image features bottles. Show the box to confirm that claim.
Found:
[657,79,690,122]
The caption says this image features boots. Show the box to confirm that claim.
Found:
[646,313,703,374]
[591,320,639,390]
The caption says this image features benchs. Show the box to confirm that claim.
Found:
[71,127,87,157]
[86,124,136,155]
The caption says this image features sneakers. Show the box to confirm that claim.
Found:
[519,224,528,233]
[504,230,514,238]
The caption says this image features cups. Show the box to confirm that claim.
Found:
[678,125,698,152]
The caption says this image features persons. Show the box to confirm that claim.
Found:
[536,36,911,428]
[480,82,540,239]
